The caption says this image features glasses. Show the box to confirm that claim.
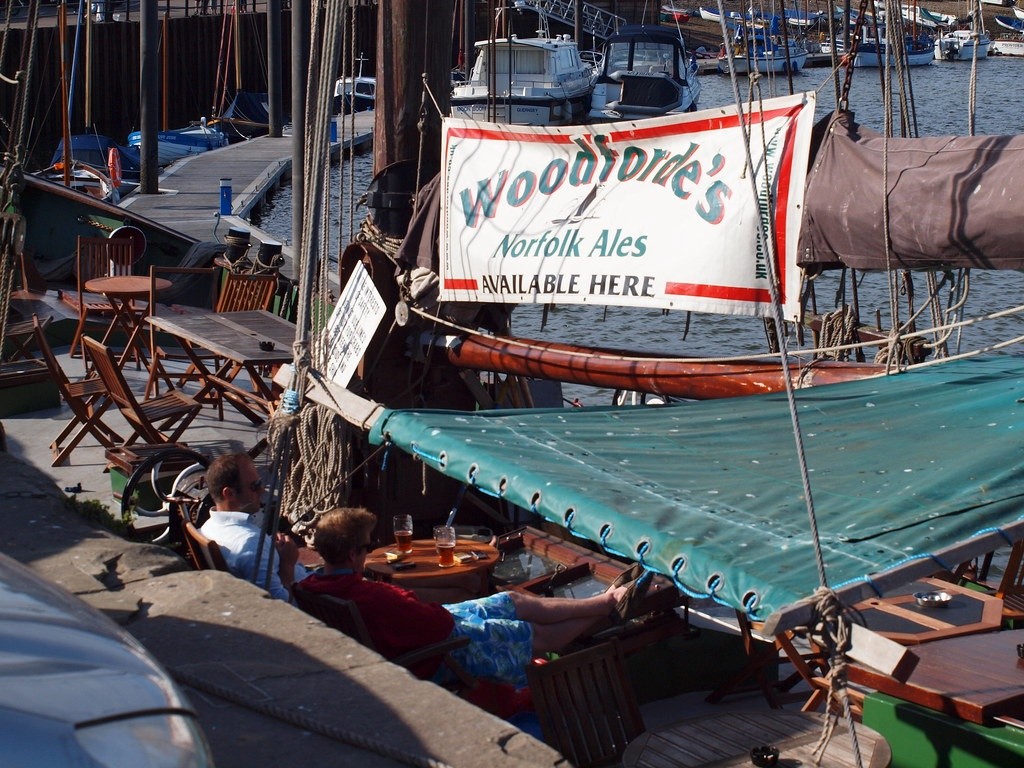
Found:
[363,543,374,553]
[240,477,263,492]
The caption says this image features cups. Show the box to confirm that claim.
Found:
[1017,644,1024,659]
[392,514,413,554]
[433,525,456,567]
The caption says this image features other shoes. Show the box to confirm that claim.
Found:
[604,562,644,594]
[608,570,653,624]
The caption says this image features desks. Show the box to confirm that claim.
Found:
[623,709,892,768]
[144,310,313,425]
[84,275,172,381]
[705,576,1003,711]
[365,539,500,602]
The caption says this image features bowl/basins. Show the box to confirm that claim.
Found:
[259,341,275,350]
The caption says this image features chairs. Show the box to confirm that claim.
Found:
[525,637,646,768]
[180,520,229,572]
[978,535,1024,625]
[292,588,476,687]
[0,227,277,474]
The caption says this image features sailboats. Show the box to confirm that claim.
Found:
[0,0,1024,768]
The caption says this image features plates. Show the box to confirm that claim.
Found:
[913,591,953,607]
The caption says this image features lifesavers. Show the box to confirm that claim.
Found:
[108,147,121,188]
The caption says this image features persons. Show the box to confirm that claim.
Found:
[193,453,308,610]
[291,507,655,691]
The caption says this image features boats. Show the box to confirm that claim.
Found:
[994,15,1024,32]
[778,8,824,28]
[874,0,958,28]
[446,2,604,129]
[659,5,690,22]
[714,8,807,75]
[836,4,884,26]
[333,79,375,111]
[588,19,702,120]
[700,6,769,31]
[819,37,846,53]
